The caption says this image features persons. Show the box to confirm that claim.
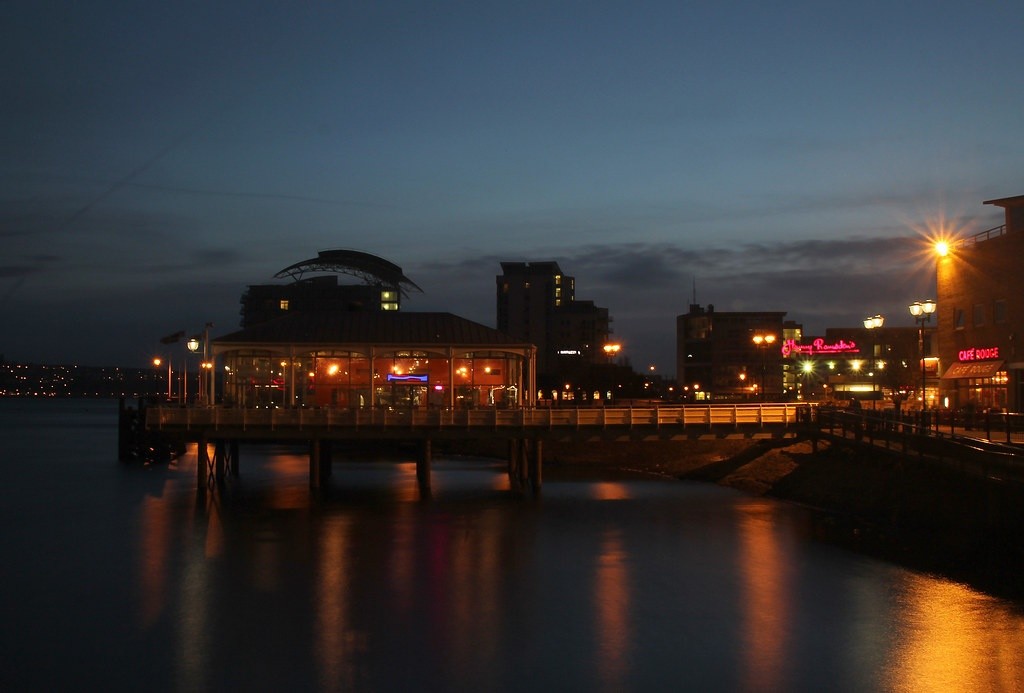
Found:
[851,395,862,409]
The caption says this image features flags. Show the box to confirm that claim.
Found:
[160,331,184,345]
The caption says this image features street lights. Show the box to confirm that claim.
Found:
[202,361,212,407]
[187,323,209,361]
[804,363,812,405]
[863,314,885,414]
[909,298,937,429]
[753,329,776,403]
[604,343,623,404]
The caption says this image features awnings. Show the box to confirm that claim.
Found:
[940,360,1005,379]
